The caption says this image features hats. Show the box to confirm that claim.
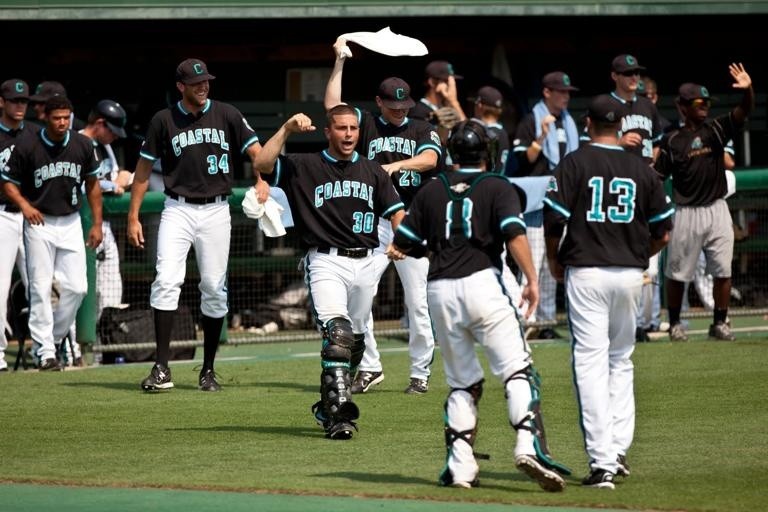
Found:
[587,94,621,123]
[378,76,415,109]
[1,79,31,100]
[542,72,578,91]
[679,83,719,102]
[176,59,215,84]
[611,54,644,72]
[426,61,462,78]
[467,86,502,108]
[28,81,66,102]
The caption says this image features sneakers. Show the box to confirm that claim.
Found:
[402,379,428,394]
[323,422,352,440]
[141,365,173,391]
[351,371,384,393]
[36,358,59,370]
[537,328,563,339]
[580,455,629,491]
[197,369,221,392]
[708,324,734,342]
[637,327,650,339]
[514,455,565,491]
[669,323,688,343]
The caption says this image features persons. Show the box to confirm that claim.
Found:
[126,59,269,390]
[324,35,442,396]
[680,248,731,331]
[542,94,676,487]
[253,104,406,437]
[392,118,566,493]
[0,55,757,369]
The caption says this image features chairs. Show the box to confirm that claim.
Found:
[7,278,38,371]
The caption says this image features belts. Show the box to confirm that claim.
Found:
[165,189,226,204]
[317,246,374,257]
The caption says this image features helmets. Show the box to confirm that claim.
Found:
[96,100,127,138]
[448,119,500,172]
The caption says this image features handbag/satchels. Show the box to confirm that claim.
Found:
[98,306,196,364]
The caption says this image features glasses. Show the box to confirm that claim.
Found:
[691,98,711,108]
